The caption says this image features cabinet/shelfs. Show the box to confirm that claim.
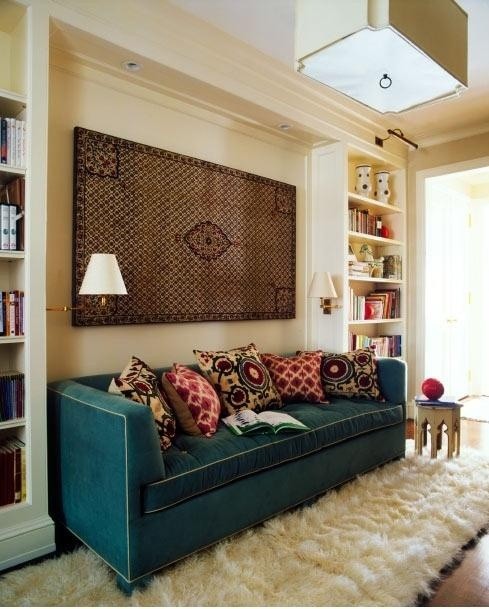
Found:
[305,140,406,362]
[1,88,47,541]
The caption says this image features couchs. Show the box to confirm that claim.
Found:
[48,350,406,597]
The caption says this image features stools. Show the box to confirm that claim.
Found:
[414,397,463,460]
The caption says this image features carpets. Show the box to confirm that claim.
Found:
[1,441,489,606]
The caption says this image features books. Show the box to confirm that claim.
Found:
[222,409,312,437]
[349,206,402,358]
[0,114,28,510]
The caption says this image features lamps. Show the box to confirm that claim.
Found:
[307,271,342,315]
[291,0,468,114]
[45,254,129,324]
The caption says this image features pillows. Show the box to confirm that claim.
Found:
[257,353,332,407]
[108,357,177,451]
[295,345,388,404]
[158,363,226,439]
[191,342,289,418]
